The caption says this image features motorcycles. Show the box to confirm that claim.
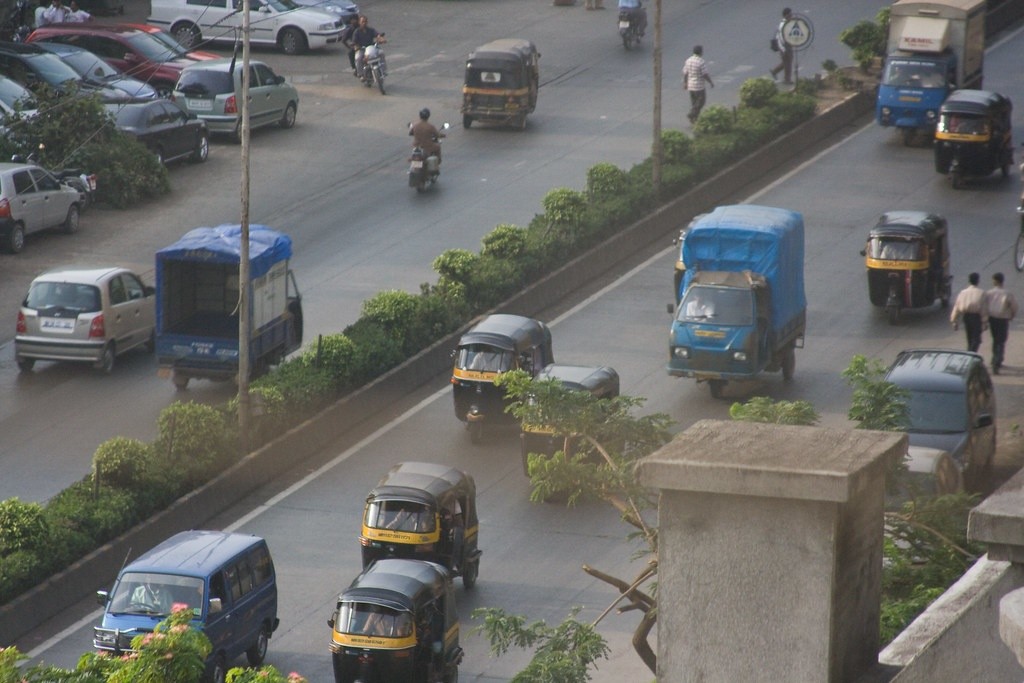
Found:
[407,121,450,194]
[860,209,955,326]
[327,460,484,683]
[353,32,389,95]
[933,88,1014,189]
[461,38,544,133]
[449,313,619,504]
[618,6,649,51]
[12,142,98,216]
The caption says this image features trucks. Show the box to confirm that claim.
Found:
[876,0,989,148]
[666,202,807,398]
[157,223,305,395]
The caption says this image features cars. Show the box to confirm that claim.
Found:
[899,446,967,500]
[169,58,300,143]
[0,162,83,254]
[16,266,157,377]
[93,95,209,166]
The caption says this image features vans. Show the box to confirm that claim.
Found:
[872,347,998,489]
[92,530,280,683]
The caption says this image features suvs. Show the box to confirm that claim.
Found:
[145,0,361,55]
[0,22,225,156]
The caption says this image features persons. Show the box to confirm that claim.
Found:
[353,601,391,640]
[680,45,715,124]
[409,108,445,165]
[341,13,387,82]
[34,0,93,28]
[948,272,988,353]
[685,289,716,324]
[469,343,506,372]
[769,7,794,85]
[131,571,174,617]
[616,0,647,38]
[987,273,1018,375]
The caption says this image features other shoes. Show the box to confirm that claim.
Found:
[782,80,793,85]
[688,112,696,124]
[770,68,778,81]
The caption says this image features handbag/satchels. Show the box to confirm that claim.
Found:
[770,30,781,51]
